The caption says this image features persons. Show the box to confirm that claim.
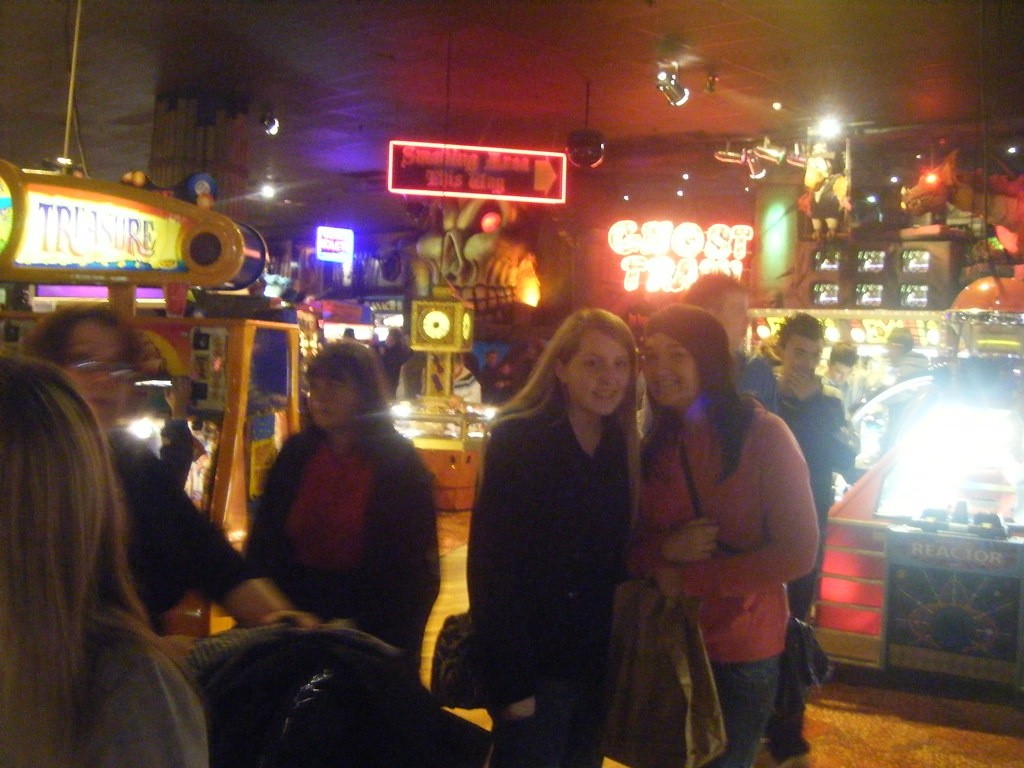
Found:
[625,307,821,767]
[687,271,778,412]
[340,326,411,397]
[820,325,930,412]
[467,306,640,768]
[733,311,845,761]
[0,302,292,768]
[245,343,440,672]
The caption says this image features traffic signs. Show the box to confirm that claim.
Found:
[388,139,568,204]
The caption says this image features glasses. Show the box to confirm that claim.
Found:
[58,350,144,389]
[300,379,357,400]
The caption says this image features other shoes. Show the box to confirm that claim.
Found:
[770,735,811,759]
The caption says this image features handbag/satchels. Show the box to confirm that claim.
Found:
[781,618,832,687]
[430,613,485,711]
[598,563,728,768]
